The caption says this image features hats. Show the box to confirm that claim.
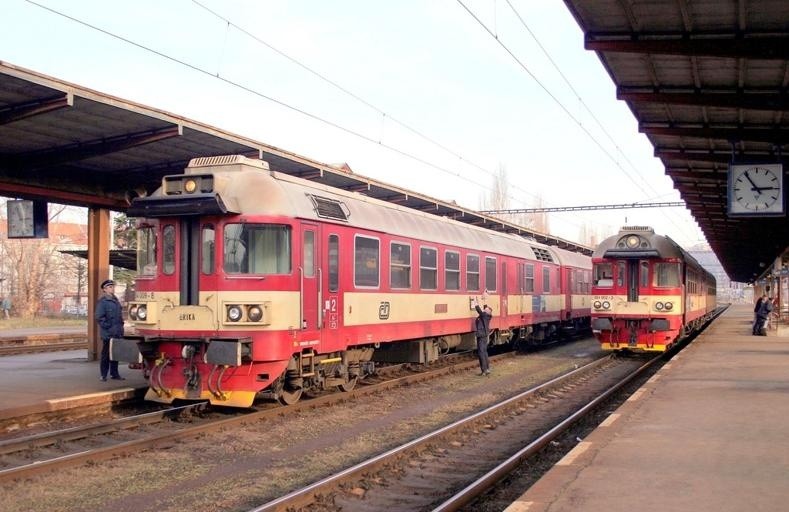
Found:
[100,280,115,289]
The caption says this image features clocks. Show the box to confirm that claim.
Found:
[727,141,786,219]
[7,199,48,238]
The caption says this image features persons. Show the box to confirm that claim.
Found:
[474,295,493,376]
[754,298,775,335]
[95,280,126,381]
[752,295,768,334]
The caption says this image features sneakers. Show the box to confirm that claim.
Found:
[110,374,126,381]
[100,376,108,381]
[480,369,490,377]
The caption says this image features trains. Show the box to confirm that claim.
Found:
[589,224,717,357]
[124,154,593,414]
